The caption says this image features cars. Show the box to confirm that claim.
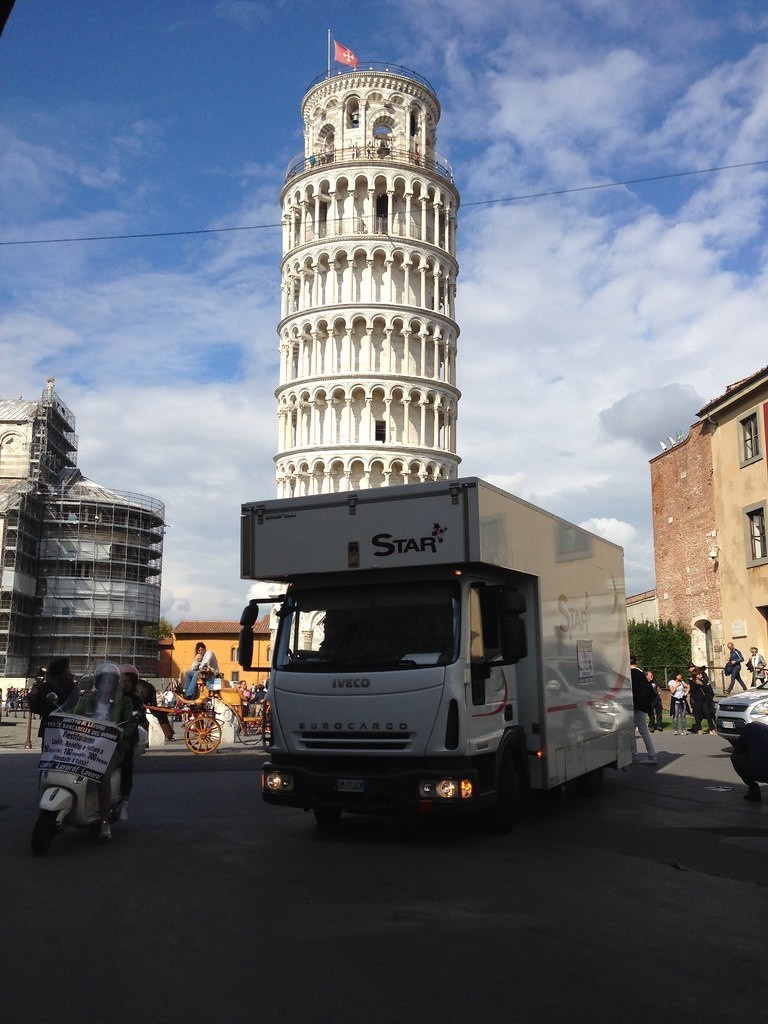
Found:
[716,680,768,747]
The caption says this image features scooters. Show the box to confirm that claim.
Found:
[27,659,150,857]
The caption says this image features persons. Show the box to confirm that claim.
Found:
[98,761,99,764]
[234,680,270,735]
[748,648,766,686]
[65,663,147,837]
[630,656,663,763]
[668,663,718,735]
[184,642,219,699]
[163,688,173,702]
[724,643,747,693]
[28,658,74,747]
[4,686,29,711]
[320,144,419,165]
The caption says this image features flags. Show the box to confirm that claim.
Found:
[334,40,358,69]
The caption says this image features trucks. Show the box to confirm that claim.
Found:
[238,476,638,839]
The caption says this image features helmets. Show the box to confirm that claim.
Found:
[94,664,120,686]
[118,664,139,683]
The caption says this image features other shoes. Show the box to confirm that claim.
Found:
[632,756,639,763]
[698,730,703,735]
[657,726,662,731]
[118,801,129,822]
[682,730,688,735]
[649,727,655,732]
[100,822,111,841]
[639,754,657,763]
[675,730,677,735]
[723,690,729,695]
[709,730,717,736]
